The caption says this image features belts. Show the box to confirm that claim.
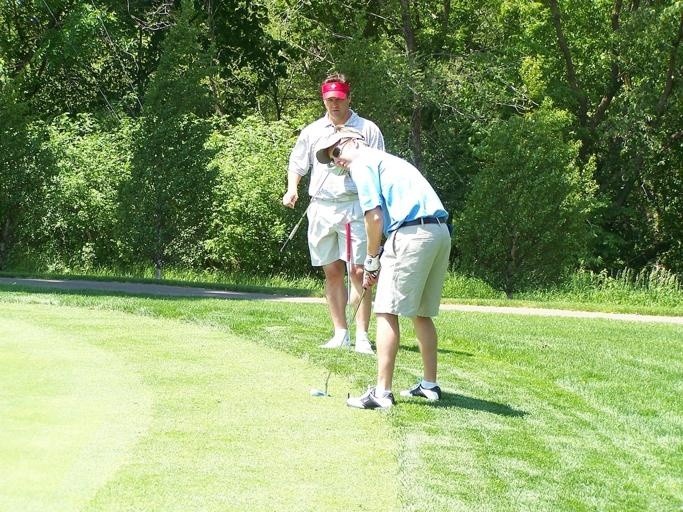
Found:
[401,217,445,227]
[336,194,358,202]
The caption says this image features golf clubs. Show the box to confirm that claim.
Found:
[311,246,384,397]
[346,223,355,350]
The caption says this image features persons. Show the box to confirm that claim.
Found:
[281,75,385,354]
[314,125,450,410]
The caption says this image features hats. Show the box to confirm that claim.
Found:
[322,81,349,101]
[316,133,365,164]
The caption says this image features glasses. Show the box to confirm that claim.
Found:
[330,139,352,164]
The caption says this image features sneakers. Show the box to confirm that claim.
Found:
[319,329,351,348]
[400,383,441,400]
[355,332,375,355]
[347,387,394,409]
